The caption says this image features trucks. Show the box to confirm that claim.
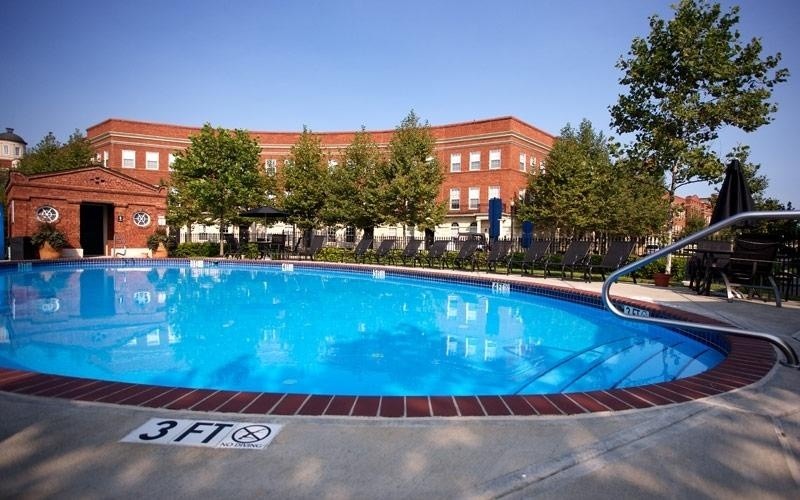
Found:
[447,232,489,251]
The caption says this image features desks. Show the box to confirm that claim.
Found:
[684,248,759,297]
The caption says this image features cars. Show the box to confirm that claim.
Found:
[644,244,659,255]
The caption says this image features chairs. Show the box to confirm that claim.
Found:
[340,235,637,284]
[224,232,325,260]
[690,239,731,291]
[707,232,784,307]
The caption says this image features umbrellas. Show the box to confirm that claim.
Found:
[709,157,762,231]
[238,204,292,243]
